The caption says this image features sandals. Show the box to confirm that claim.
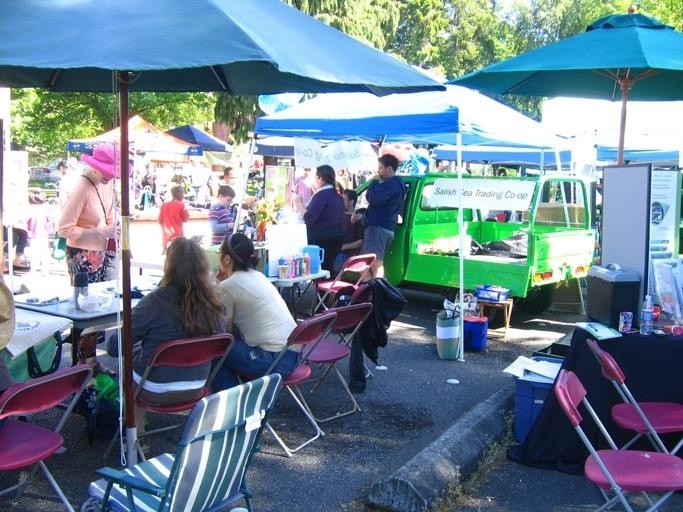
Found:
[87,362,117,377]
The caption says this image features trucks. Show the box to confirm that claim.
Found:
[342,173,596,327]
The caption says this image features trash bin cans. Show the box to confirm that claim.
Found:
[435,309,461,361]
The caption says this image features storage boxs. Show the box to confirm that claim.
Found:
[587,263,640,328]
[464,320,488,352]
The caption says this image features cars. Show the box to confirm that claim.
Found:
[28,160,61,182]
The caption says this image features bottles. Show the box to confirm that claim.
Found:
[276,251,310,281]
[264,250,269,277]
[639,294,654,336]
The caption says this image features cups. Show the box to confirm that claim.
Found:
[495,213,507,223]
[618,311,633,335]
[107,220,121,257]
[73,272,89,311]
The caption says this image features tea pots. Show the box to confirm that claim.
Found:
[302,244,326,275]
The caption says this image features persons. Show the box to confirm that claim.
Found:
[107,234,230,456]
[350,153,406,283]
[292,164,347,313]
[434,159,472,175]
[204,234,302,454]
[55,142,133,377]
[2,151,369,278]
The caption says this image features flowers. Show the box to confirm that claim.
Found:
[249,198,277,229]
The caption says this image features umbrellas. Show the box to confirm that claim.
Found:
[0,1,448,469]
[441,6,683,166]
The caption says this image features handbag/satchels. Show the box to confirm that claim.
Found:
[65,238,119,286]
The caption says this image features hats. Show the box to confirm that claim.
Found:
[81,144,133,179]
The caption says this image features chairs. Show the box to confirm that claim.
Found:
[555,369,683,512]
[587,338,683,510]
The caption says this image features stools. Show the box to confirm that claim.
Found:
[478,299,513,342]
